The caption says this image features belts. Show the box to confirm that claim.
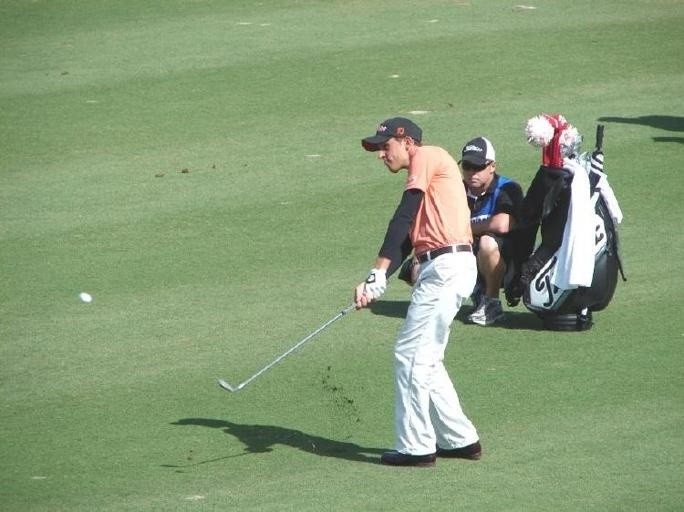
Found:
[417,244,472,264]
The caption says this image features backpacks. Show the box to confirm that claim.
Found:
[508,165,618,318]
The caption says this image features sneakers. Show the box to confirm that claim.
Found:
[467,295,505,325]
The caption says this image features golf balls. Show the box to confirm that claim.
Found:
[80,292,92,302]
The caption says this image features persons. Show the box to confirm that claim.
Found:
[397,136,523,326]
[354,118,481,465]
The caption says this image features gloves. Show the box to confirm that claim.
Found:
[362,268,387,298]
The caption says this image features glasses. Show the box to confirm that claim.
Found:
[461,161,493,171]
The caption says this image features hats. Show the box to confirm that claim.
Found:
[361,117,422,151]
[457,136,496,165]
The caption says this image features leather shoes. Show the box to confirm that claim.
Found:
[436,442,482,461]
[381,451,437,466]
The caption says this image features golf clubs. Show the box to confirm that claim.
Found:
[218,303,358,392]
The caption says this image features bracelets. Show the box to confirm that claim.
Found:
[409,261,421,269]
